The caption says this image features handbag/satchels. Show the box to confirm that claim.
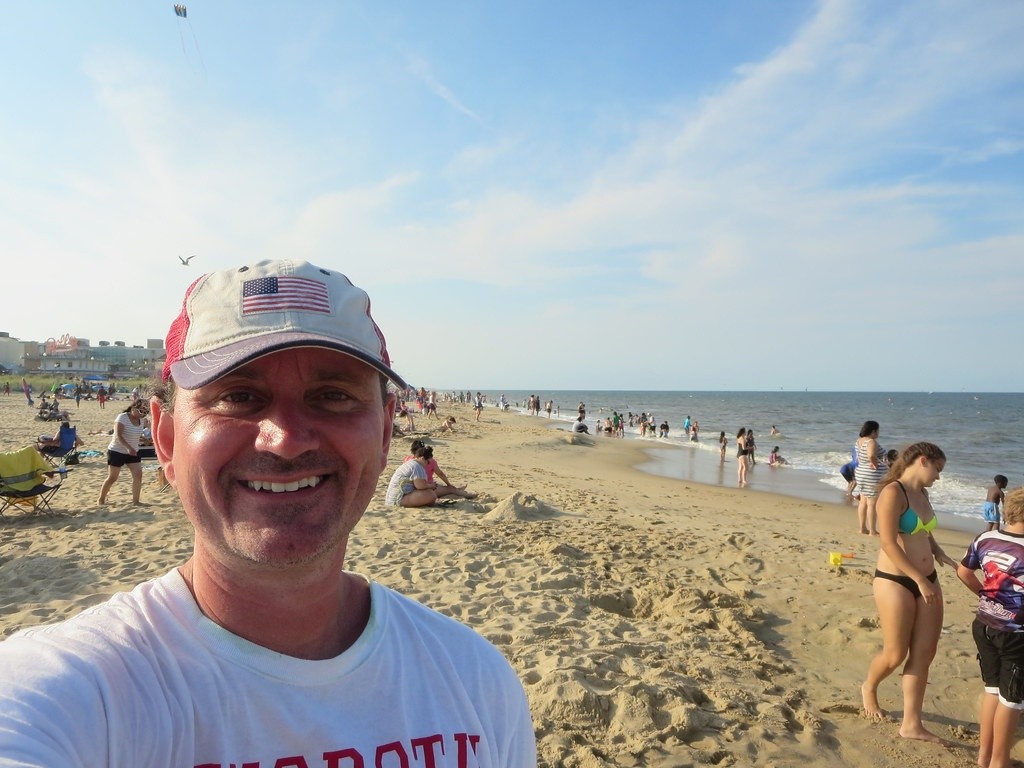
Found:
[65,452,80,465]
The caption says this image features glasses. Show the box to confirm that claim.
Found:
[424,458,430,464]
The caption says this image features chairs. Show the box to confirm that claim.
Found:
[41,426,78,467]
[0,444,73,526]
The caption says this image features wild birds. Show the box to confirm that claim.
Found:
[178,255,196,265]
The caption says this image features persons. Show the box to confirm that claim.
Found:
[595,411,669,437]
[36,377,148,422]
[96,399,150,505]
[578,402,585,419]
[573,416,592,435]
[720,431,727,454]
[857,442,959,742]
[0,261,543,768]
[985,475,1008,531]
[33,421,84,458]
[769,446,782,464]
[139,418,154,446]
[956,486,1024,768]
[385,440,479,507]
[838,419,900,535]
[4,382,10,396]
[391,386,554,435]
[737,427,757,483]
[771,426,776,434]
[684,416,699,441]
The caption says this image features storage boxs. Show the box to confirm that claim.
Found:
[159,469,168,485]
[139,442,157,460]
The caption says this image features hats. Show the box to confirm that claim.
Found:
[163,259,408,390]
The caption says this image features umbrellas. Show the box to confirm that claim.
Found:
[22,377,34,405]
